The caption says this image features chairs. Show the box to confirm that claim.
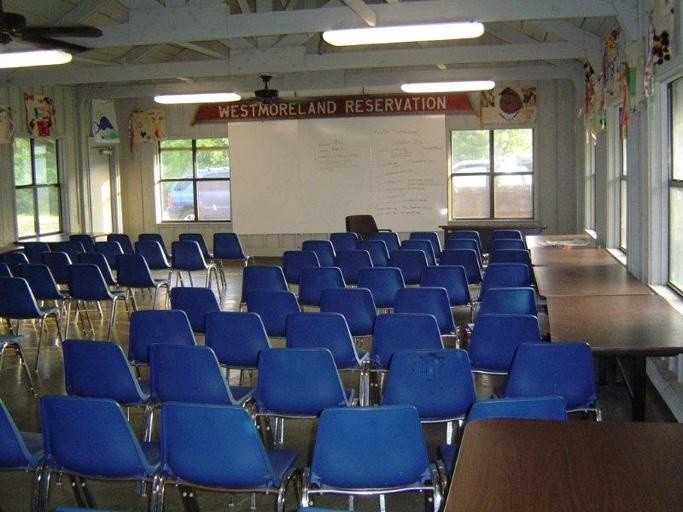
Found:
[1,216,608,512]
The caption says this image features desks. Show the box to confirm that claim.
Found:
[542,294,679,423]
[438,413,682,511]
[527,262,658,300]
[526,245,621,263]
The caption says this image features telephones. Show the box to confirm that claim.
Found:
[228,111,449,237]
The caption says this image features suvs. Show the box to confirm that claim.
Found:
[450,159,531,219]
[166,166,230,222]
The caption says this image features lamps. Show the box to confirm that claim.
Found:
[150,89,243,111]
[321,20,488,48]
[0,44,72,69]
[396,78,497,94]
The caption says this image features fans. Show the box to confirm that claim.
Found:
[0,0,105,55]
[228,73,314,108]
